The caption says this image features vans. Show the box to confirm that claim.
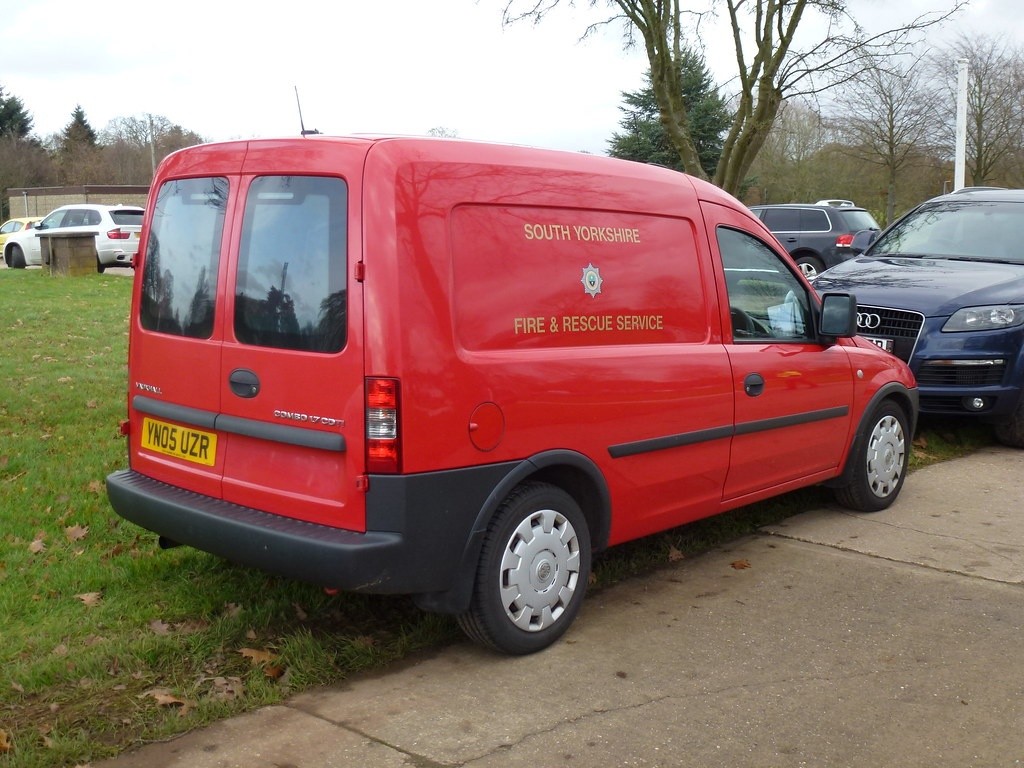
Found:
[106,135,920,656]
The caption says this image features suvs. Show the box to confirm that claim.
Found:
[785,184,1024,451]
[716,199,883,288]
[3,203,146,274]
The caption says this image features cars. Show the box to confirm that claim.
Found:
[0,217,45,254]
